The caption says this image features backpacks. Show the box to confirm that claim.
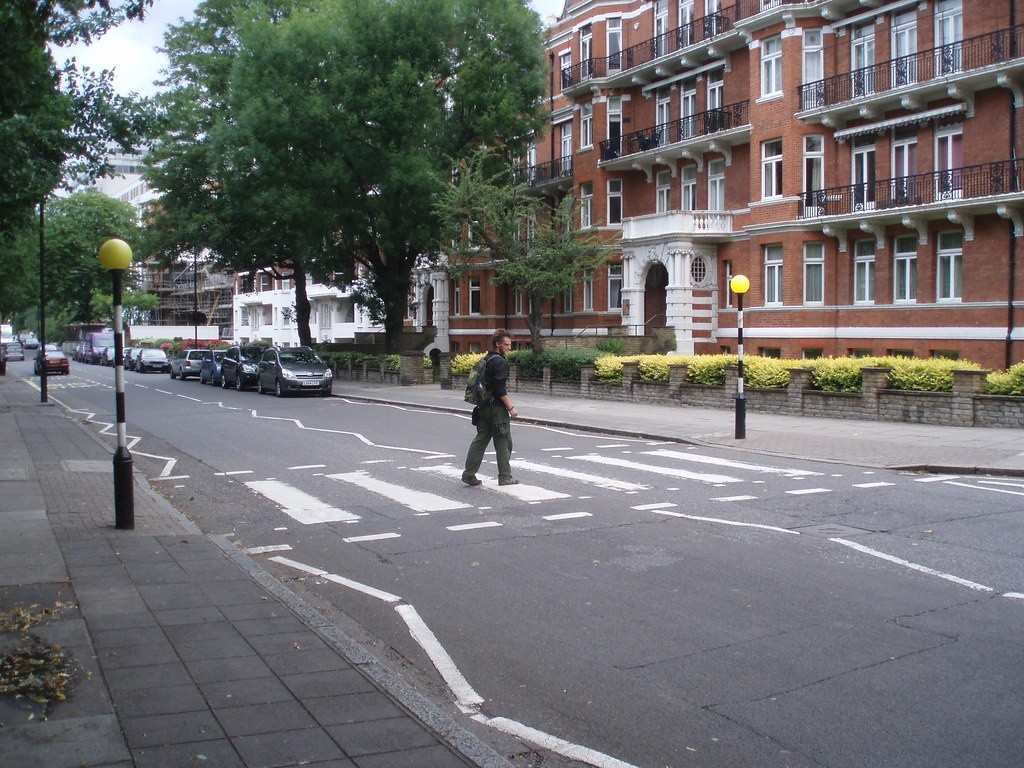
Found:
[464,354,505,407]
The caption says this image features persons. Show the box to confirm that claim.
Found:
[461,328,519,486]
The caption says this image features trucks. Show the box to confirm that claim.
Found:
[0,324,12,344]
[82,332,113,365]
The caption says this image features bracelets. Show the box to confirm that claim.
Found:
[508,406,514,411]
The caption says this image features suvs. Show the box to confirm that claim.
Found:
[257,345,333,398]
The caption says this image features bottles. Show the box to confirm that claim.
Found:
[478,381,483,389]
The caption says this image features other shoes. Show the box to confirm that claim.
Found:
[499,477,520,485]
[462,470,482,486]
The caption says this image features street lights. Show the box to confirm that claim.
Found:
[731,275,750,439]
[99,239,135,529]
[40,194,52,403]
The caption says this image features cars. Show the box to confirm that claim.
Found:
[17,334,32,345]
[200,350,228,386]
[221,346,268,391]
[3,344,24,361]
[112,348,131,368]
[98,347,114,367]
[37,344,57,355]
[33,351,70,376]
[123,348,143,371]
[168,349,210,380]
[135,349,171,374]
[72,342,86,363]
[23,338,39,349]
